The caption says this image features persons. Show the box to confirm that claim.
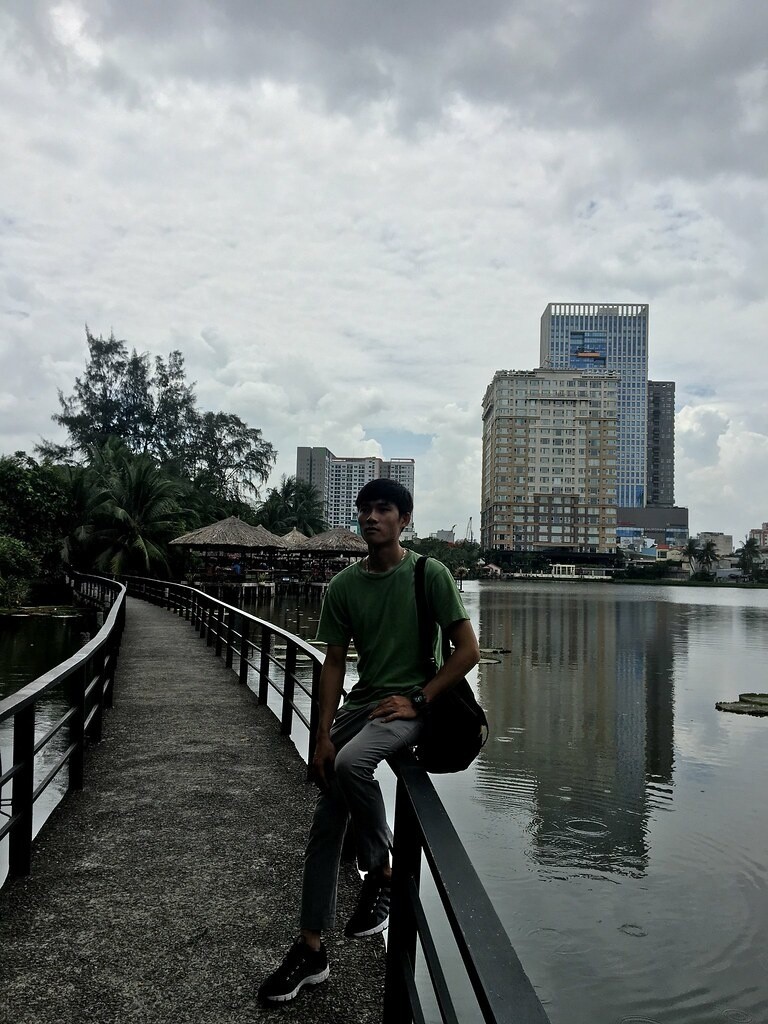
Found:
[233,562,241,574]
[214,564,220,570]
[254,479,481,1002]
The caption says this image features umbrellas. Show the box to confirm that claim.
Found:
[257,523,310,551]
[298,525,369,557]
[167,516,288,552]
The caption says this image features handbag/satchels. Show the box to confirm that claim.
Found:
[413,555,491,775]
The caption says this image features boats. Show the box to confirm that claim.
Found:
[501,562,615,582]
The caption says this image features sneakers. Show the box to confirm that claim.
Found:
[258,939,330,1002]
[346,867,394,937]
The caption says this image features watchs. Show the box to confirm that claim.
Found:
[407,691,428,711]
